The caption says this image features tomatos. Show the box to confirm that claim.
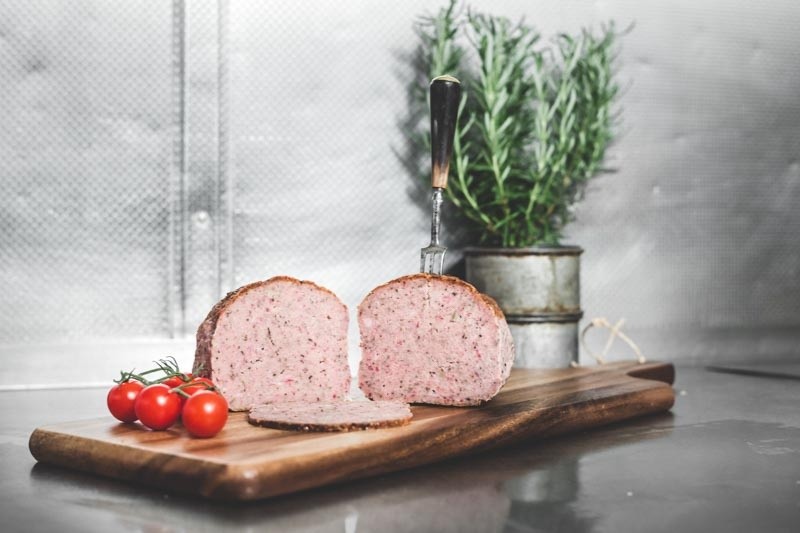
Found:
[107,372,229,438]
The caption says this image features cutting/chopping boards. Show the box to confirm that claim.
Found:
[28,360,677,503]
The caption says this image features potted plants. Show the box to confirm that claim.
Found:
[401,0,642,368]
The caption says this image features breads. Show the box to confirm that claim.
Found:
[358,274,515,407]
[191,276,349,412]
[247,401,412,432]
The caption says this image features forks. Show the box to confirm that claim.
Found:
[419,75,461,274]
